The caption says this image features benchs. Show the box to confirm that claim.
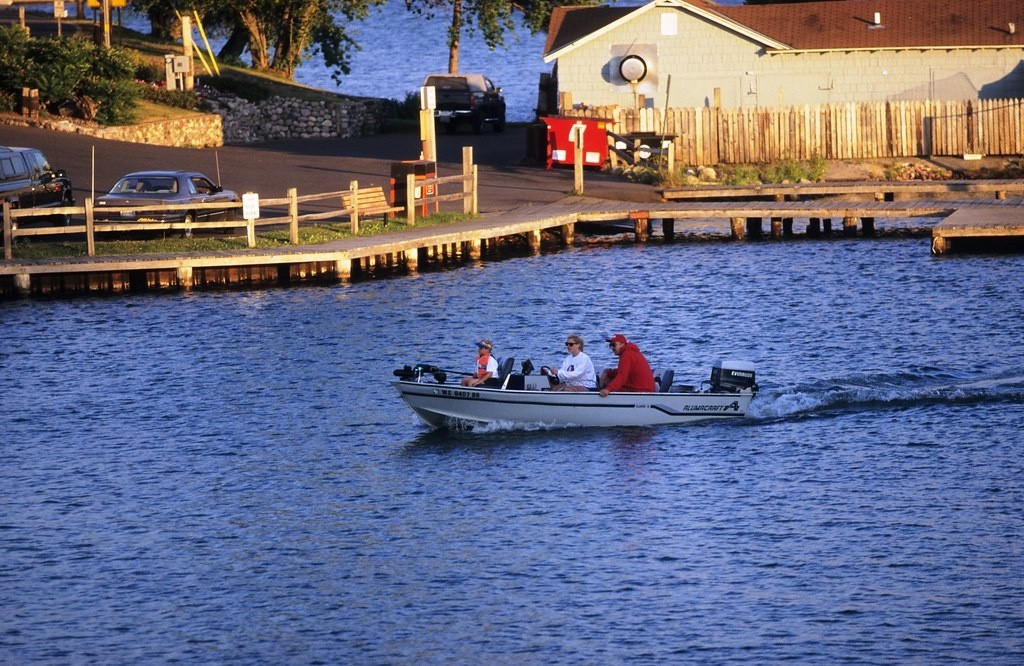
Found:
[340,186,405,227]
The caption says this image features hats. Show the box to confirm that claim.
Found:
[474,340,492,349]
[606,334,627,343]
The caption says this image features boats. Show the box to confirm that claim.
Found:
[388,357,760,431]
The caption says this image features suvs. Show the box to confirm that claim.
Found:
[0,145,76,241]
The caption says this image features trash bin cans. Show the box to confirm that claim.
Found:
[539,115,614,169]
[390,159,440,219]
[526,124,545,158]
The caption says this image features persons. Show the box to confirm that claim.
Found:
[598,333,655,397]
[461,339,500,387]
[543,335,597,392]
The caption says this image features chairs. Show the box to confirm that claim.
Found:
[136,182,151,191]
[475,358,515,390]
[658,369,674,393]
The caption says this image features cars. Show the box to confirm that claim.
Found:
[95,170,241,238]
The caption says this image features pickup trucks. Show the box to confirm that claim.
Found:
[415,74,505,134]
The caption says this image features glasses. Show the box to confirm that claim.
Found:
[564,342,577,346]
[478,345,485,349]
[609,343,616,348]
